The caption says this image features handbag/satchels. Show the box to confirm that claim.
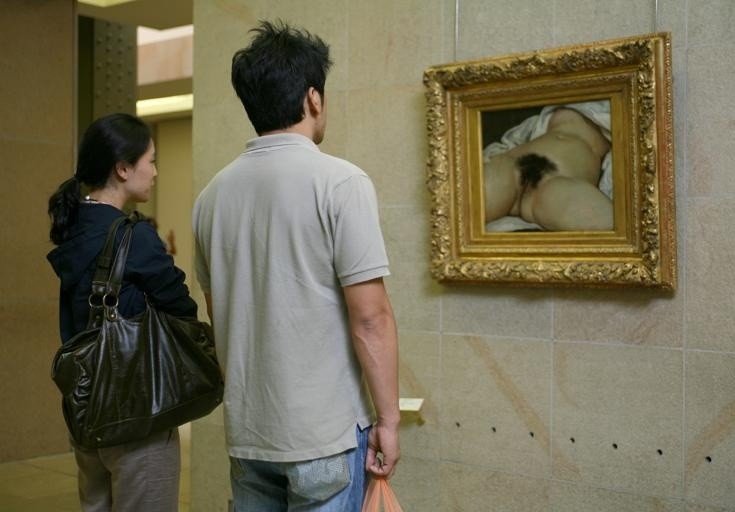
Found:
[51,211,224,451]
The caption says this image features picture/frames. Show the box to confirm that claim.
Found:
[421,34,677,297]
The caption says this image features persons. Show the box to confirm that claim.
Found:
[478,105,620,238]
[48,111,199,510]
[187,17,404,512]
